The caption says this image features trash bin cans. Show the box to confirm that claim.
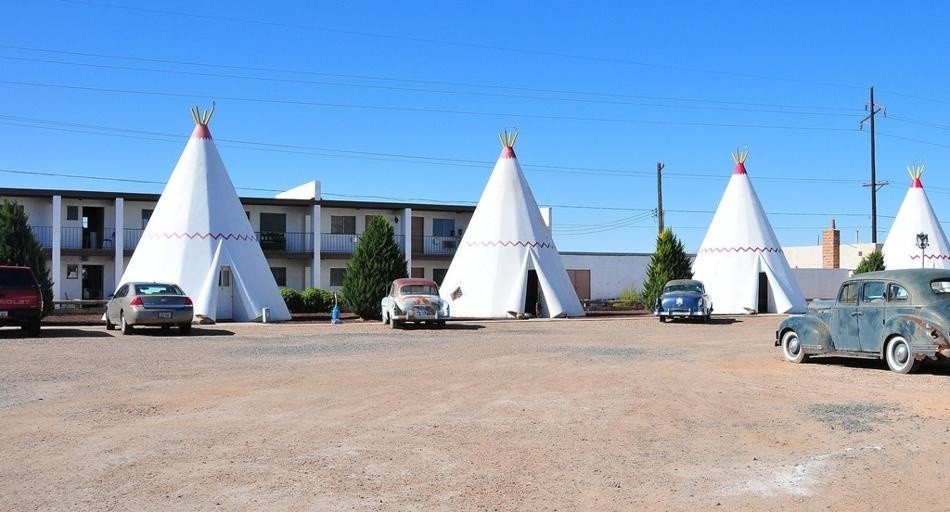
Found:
[262,308,270,323]
[112,232,115,248]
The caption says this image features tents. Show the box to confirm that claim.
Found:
[99,97,292,324]
[870,159,950,295]
[438,129,587,320]
[690,146,809,315]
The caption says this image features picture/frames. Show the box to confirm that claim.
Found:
[66,263,78,279]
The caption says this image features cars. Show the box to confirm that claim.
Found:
[105,281,193,335]
[654,279,713,323]
[1,266,44,336]
[380,278,451,329]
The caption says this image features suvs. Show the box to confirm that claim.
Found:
[774,268,950,374]
